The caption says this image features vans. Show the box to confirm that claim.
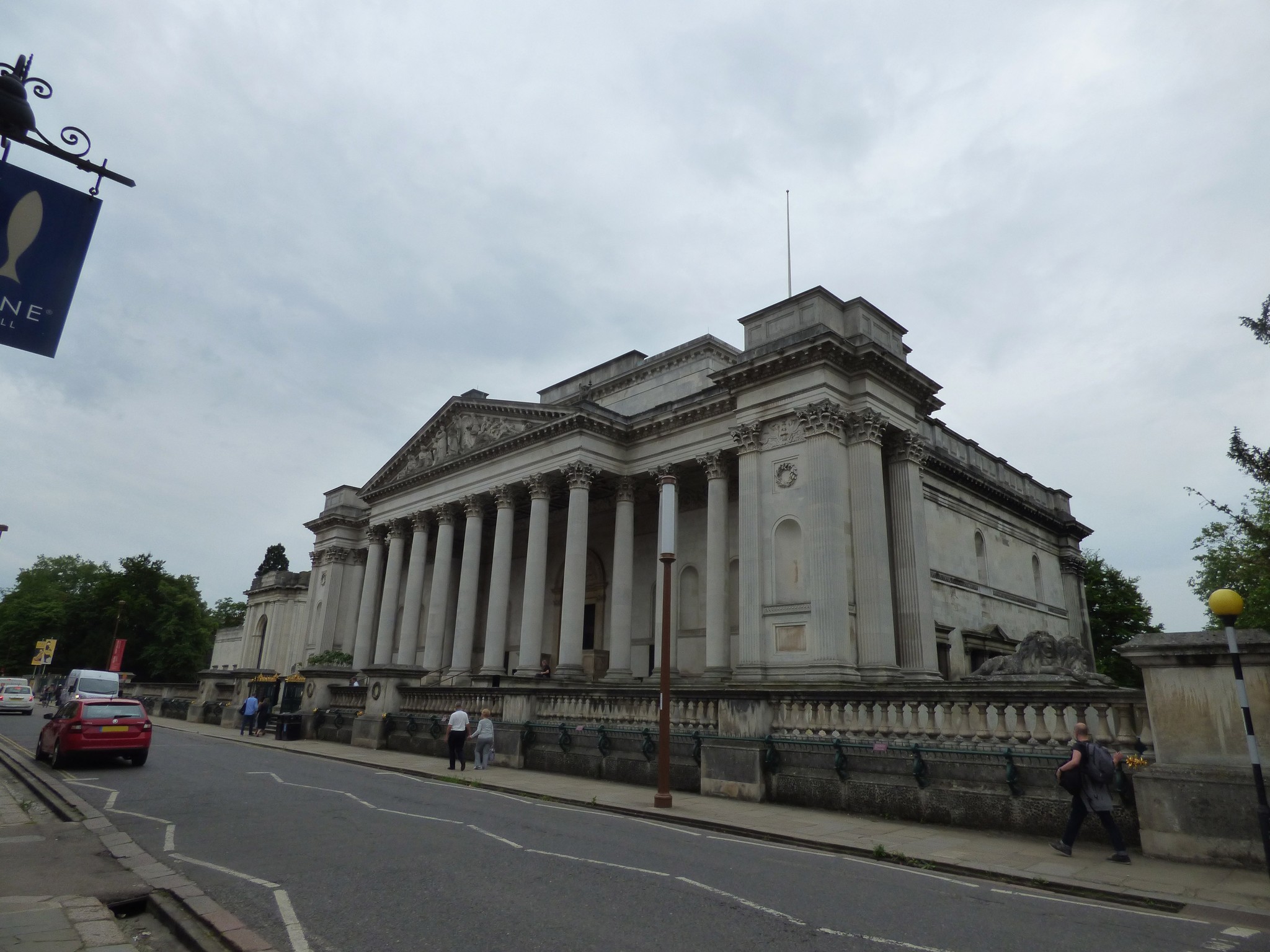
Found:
[55,669,119,708]
[0,678,28,692]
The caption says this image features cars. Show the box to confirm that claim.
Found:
[35,696,153,769]
[0,685,35,715]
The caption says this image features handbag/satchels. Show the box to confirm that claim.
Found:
[489,745,495,761]
[239,698,248,715]
[1055,762,1083,795]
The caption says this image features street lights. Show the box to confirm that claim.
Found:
[1208,588,1269,877]
[653,474,677,808]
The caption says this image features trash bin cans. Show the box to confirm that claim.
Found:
[275,713,303,741]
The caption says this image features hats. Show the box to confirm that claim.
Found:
[51,683,54,685]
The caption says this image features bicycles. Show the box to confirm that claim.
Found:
[41,692,53,707]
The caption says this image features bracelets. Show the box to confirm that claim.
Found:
[1059,767,1063,772]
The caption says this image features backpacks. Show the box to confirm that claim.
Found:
[1077,740,1116,785]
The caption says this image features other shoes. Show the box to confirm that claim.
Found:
[256,734,259,737]
[262,732,266,735]
[1049,839,1072,857]
[447,766,455,770]
[1106,853,1131,864]
[461,759,465,771]
[474,765,482,770]
[249,733,254,736]
[240,733,244,736]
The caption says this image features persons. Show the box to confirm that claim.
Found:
[540,659,551,678]
[33,641,52,663]
[352,677,359,686]
[42,683,63,706]
[254,697,272,737]
[1050,723,1132,864]
[444,701,471,771]
[467,709,495,770]
[240,692,258,736]
